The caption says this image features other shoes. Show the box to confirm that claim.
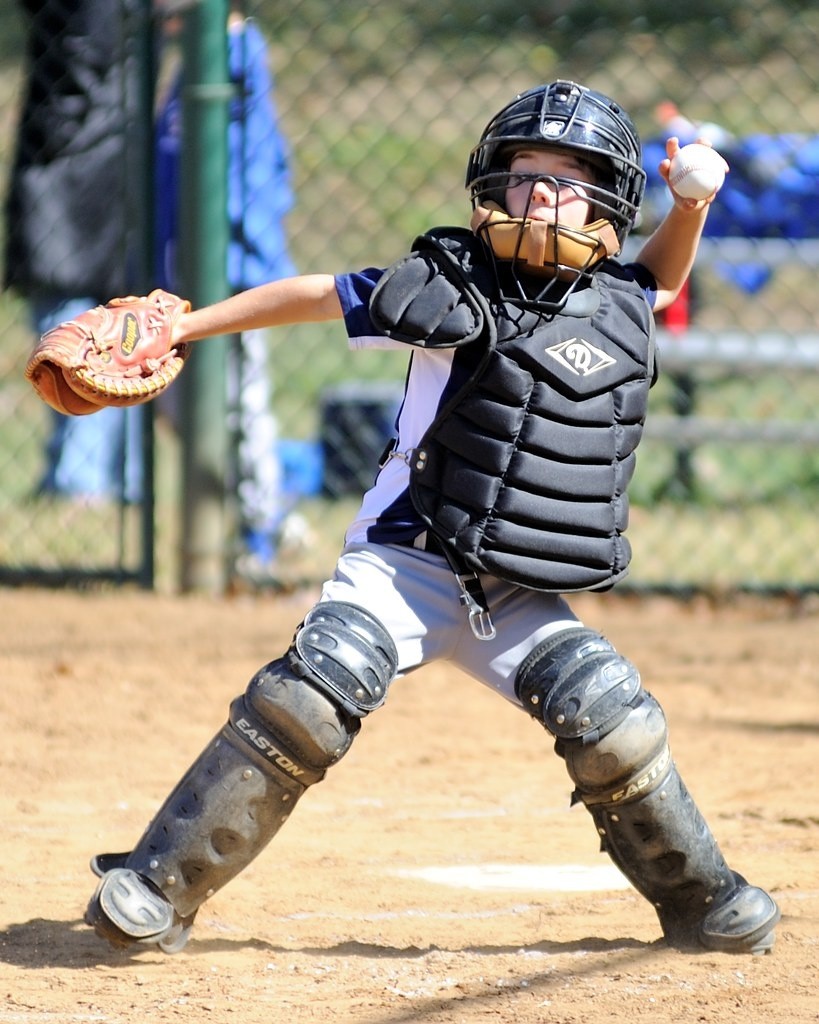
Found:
[661,868,781,953]
[83,867,200,952]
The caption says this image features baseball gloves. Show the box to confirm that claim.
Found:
[24,289,193,416]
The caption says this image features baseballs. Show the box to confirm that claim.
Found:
[669,144,725,199]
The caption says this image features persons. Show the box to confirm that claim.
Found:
[2,0,300,564]
[24,80,782,953]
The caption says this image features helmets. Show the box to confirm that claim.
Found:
[465,79,641,235]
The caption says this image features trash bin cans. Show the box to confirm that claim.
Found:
[317,393,400,507]
